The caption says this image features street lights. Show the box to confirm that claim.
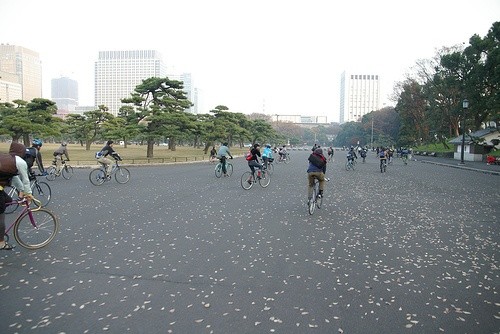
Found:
[460,97,469,163]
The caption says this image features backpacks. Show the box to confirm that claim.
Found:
[246,151,253,160]
[380,151,384,157]
[309,153,326,168]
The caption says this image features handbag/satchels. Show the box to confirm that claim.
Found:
[96,152,103,159]
[54,152,59,156]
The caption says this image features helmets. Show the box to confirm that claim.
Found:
[32,139,42,146]
[266,144,272,148]
[61,142,67,146]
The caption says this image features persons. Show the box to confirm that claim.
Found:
[53,142,69,172]
[262,144,273,171]
[246,143,264,185]
[273,143,418,170]
[0,141,33,249]
[306,148,327,205]
[217,142,232,176]
[20,138,45,180]
[95,140,120,176]
[210,146,218,159]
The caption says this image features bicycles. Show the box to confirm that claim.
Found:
[4,171,52,215]
[308,174,325,215]
[214,157,233,178]
[89,159,131,186]
[0,193,59,251]
[241,163,271,191]
[209,148,415,173]
[46,159,73,181]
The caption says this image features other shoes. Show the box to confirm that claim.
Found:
[102,177,105,180]
[0,242,13,250]
[319,193,324,198]
[224,173,228,177]
[258,176,262,179]
[307,200,312,205]
[107,175,111,178]
[54,173,60,176]
[347,154,352,157]
[247,179,251,184]
